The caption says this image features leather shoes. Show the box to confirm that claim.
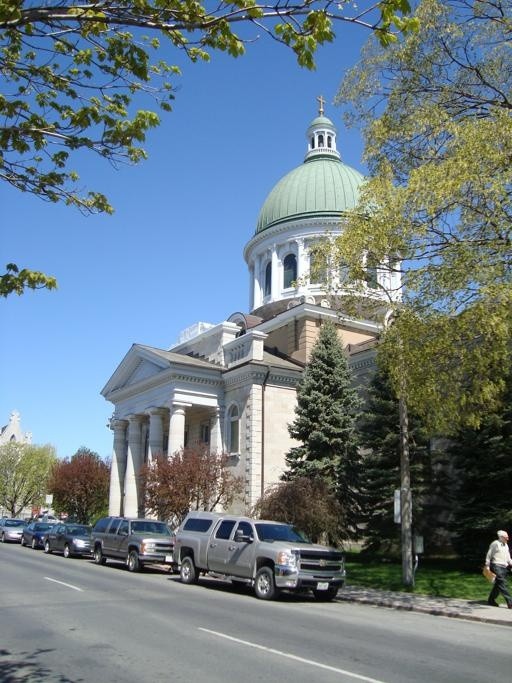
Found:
[488,600,498,605]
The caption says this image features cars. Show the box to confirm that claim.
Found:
[1,519,92,557]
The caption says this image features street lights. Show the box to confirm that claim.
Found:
[353,267,414,592]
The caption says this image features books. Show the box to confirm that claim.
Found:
[481,567,497,585]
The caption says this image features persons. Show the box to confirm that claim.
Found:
[485,529,512,610]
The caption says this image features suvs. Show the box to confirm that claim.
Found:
[173,510,347,602]
[90,516,175,572]
